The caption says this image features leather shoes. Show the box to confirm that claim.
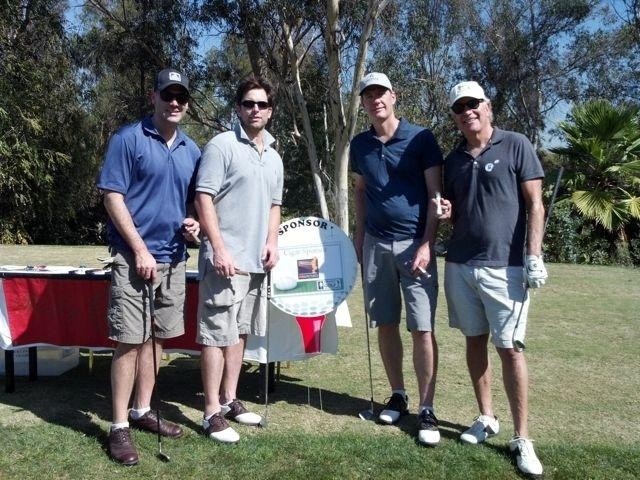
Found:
[107,428,139,466]
[127,410,184,438]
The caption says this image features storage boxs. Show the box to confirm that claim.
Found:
[0,344,80,378]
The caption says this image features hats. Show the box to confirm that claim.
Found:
[357,72,394,96]
[447,81,489,109]
[155,68,190,96]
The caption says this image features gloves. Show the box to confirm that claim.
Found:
[523,255,548,288]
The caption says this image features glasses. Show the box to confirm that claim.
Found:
[158,90,190,106]
[450,99,484,114]
[237,99,270,111]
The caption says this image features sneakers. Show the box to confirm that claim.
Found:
[460,412,500,444]
[201,397,262,442]
[417,408,441,446]
[508,435,543,475]
[379,393,409,424]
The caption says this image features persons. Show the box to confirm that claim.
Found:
[192,79,285,444]
[431,81,548,479]
[350,72,443,446]
[96,66,201,466]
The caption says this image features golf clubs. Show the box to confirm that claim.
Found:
[259,255,271,427]
[148,278,170,462]
[512,166,564,352]
[359,256,374,421]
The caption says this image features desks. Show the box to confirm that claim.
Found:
[1,265,288,401]
